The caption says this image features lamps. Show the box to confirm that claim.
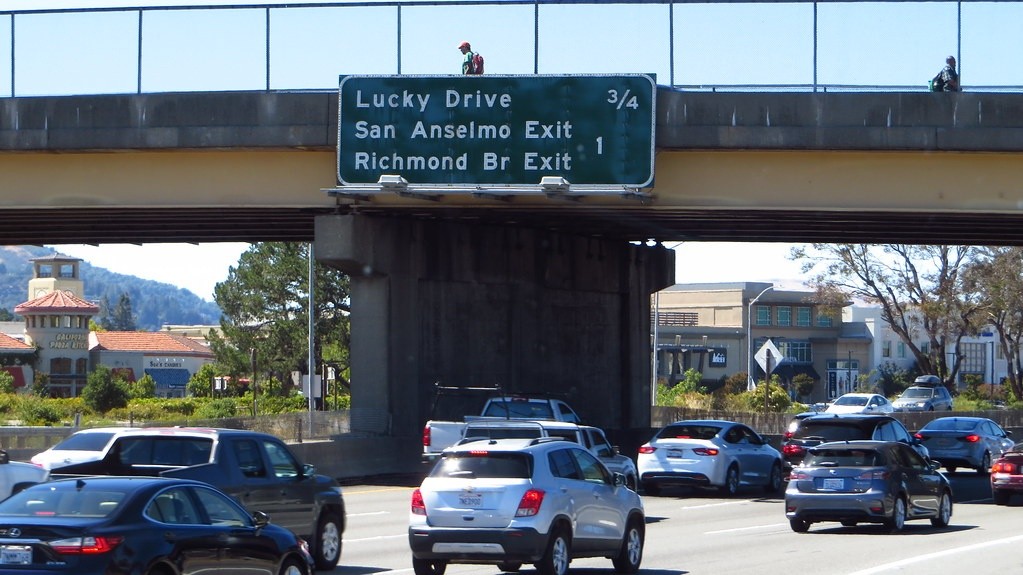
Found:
[377,174,407,188]
[539,176,572,190]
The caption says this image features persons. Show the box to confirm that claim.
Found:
[941,56,962,92]
[457,42,474,74]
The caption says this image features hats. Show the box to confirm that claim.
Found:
[458,42,471,49]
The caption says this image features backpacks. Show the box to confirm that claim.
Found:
[470,52,484,74]
[929,66,956,92]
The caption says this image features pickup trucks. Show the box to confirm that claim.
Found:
[50,427,346,569]
[421,378,583,487]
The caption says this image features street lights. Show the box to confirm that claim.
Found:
[747,283,786,392]
[988,340,993,396]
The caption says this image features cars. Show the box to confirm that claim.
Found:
[784,438,953,534]
[26,428,142,471]
[637,420,784,495]
[0,476,316,575]
[914,415,1016,474]
[825,392,893,413]
[989,438,1023,504]
[0,446,55,500]
[782,413,815,438]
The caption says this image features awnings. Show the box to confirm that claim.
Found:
[757,363,821,382]
[145,369,192,389]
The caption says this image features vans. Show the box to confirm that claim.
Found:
[783,413,929,487]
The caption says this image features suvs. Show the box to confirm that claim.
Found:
[408,432,646,575]
[892,374,953,412]
[459,418,637,494]
